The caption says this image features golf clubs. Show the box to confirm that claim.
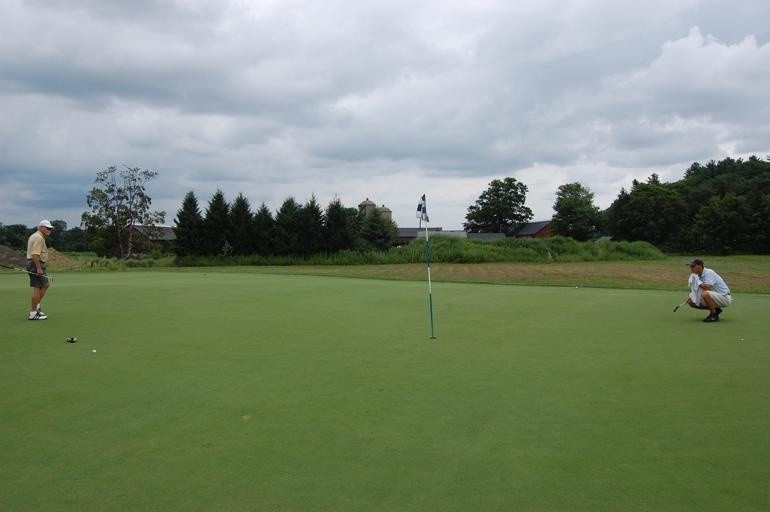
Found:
[0,265,53,282]
[673,301,688,312]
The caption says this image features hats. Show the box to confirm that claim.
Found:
[39,219,54,229]
[686,258,704,266]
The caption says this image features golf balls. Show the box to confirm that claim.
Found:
[576,286,578,288]
[92,349,97,354]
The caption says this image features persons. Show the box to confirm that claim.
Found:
[686,259,734,322]
[27,220,53,320]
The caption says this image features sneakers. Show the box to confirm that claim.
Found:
[28,310,48,320]
[702,307,722,322]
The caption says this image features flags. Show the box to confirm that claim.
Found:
[416,194,429,222]
